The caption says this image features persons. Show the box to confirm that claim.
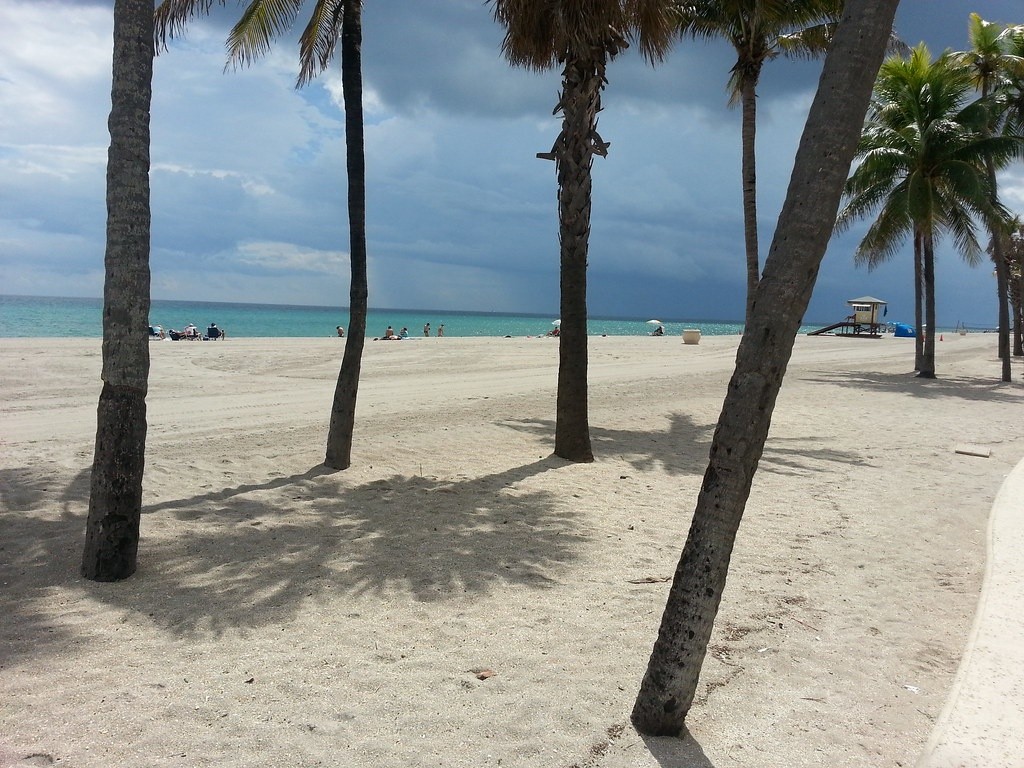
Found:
[386,326,393,339]
[424,323,430,336]
[336,326,344,337]
[169,329,185,337]
[401,328,407,337]
[149,325,164,339]
[438,325,444,336]
[187,324,197,340]
[210,323,217,340]
[654,326,662,335]
[546,327,558,336]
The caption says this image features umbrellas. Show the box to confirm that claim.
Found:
[646,319,662,333]
[552,319,561,325]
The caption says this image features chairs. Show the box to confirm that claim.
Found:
[169,332,185,340]
[149,327,159,338]
[184,326,200,341]
[207,327,225,341]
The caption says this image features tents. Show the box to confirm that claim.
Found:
[895,324,916,337]
